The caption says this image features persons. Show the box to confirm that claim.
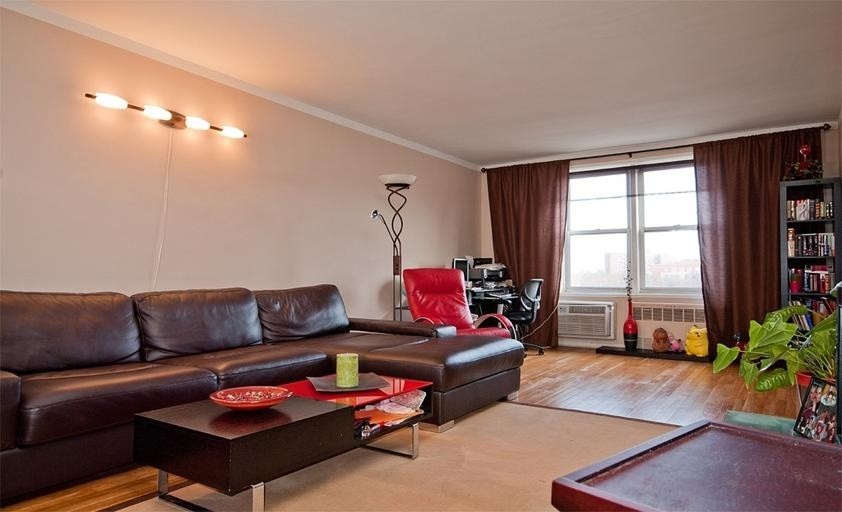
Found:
[797,384,838,443]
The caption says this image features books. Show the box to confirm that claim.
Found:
[786,198,835,332]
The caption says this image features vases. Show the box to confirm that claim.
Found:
[623,302,638,349]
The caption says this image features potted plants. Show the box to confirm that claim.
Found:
[713,281,842,391]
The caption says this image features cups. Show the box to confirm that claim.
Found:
[465,281,472,288]
[335,352,359,388]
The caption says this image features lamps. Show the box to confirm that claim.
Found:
[372,173,416,322]
[86,92,247,140]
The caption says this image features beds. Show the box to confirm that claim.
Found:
[256,283,526,425]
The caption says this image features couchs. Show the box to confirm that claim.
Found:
[0,288,329,504]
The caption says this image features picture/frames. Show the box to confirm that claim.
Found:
[793,376,838,443]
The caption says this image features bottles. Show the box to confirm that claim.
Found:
[787,228,796,257]
[790,273,802,293]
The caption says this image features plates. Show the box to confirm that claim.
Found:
[208,384,292,414]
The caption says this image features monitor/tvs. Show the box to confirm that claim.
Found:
[452,258,467,281]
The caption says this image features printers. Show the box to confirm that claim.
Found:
[465,256,507,281]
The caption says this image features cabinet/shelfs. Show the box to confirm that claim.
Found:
[780,177,842,370]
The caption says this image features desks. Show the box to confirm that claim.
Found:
[724,410,841,443]
[472,296,520,327]
[552,419,842,512]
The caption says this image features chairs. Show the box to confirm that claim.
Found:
[403,269,517,340]
[503,279,544,355]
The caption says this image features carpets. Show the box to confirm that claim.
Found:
[97,398,682,512]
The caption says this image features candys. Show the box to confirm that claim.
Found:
[216,390,278,401]
